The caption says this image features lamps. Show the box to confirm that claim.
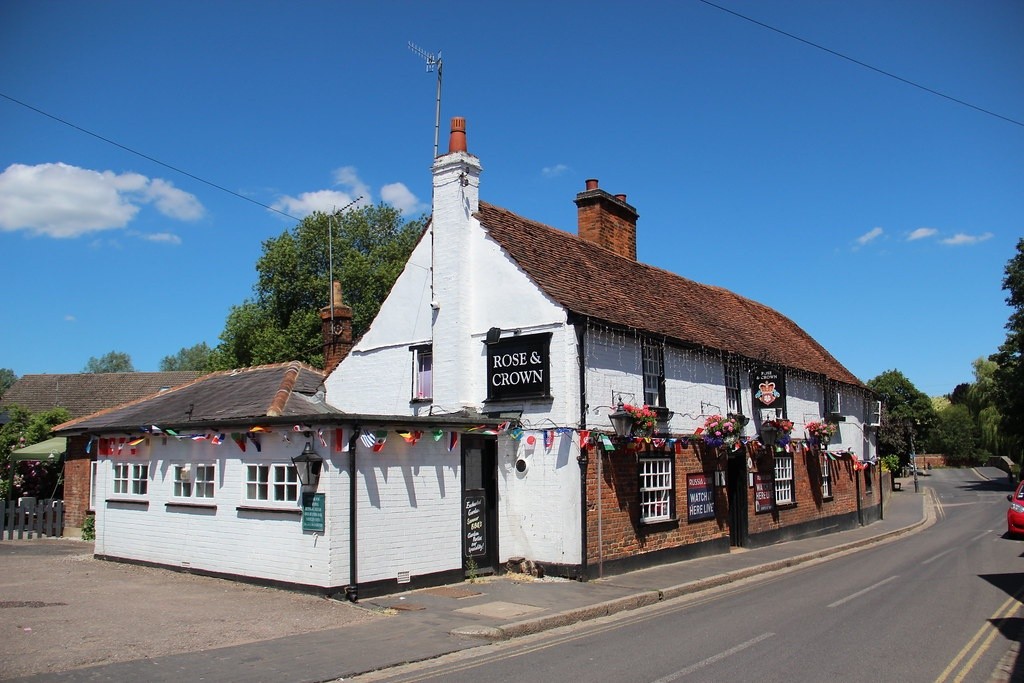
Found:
[761,418,777,446]
[822,433,830,450]
[608,396,634,442]
[291,431,323,486]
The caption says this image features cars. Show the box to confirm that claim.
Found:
[1006,481,1024,536]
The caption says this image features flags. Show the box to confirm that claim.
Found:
[231,426,269,452]
[525,431,537,448]
[824,451,858,461]
[556,428,615,450]
[775,441,810,453]
[461,422,523,439]
[357,429,375,447]
[86,437,145,455]
[731,434,760,453]
[290,424,327,447]
[396,429,457,452]
[373,431,387,452]
[543,429,554,447]
[175,433,226,445]
[141,425,177,436]
[335,428,350,451]
[626,428,704,454]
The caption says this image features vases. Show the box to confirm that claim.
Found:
[632,425,653,438]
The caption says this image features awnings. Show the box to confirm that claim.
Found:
[8,437,67,500]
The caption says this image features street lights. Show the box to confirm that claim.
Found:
[906,421,919,493]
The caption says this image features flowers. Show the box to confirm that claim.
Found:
[703,415,744,446]
[762,419,795,446]
[806,419,837,444]
[610,402,659,436]
